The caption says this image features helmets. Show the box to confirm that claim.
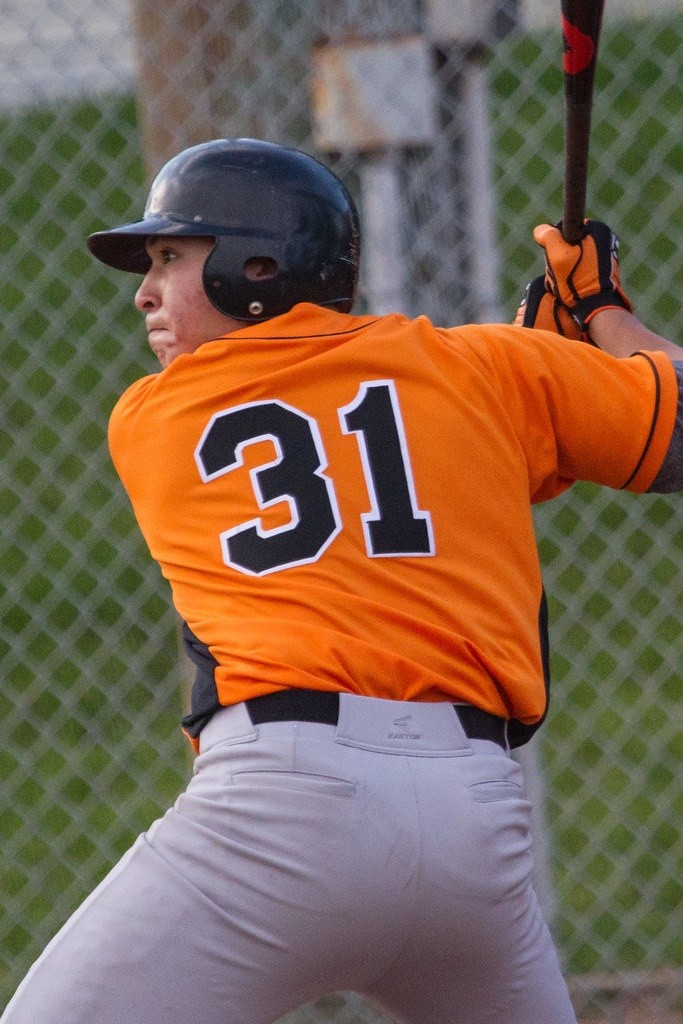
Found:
[85,138,359,324]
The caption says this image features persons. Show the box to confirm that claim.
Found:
[1,137,682,1024]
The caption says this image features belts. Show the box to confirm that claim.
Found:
[244,689,508,749]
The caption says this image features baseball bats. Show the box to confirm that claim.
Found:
[562,1,609,236]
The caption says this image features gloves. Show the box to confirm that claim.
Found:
[533,220,632,342]
[515,274,587,344]
